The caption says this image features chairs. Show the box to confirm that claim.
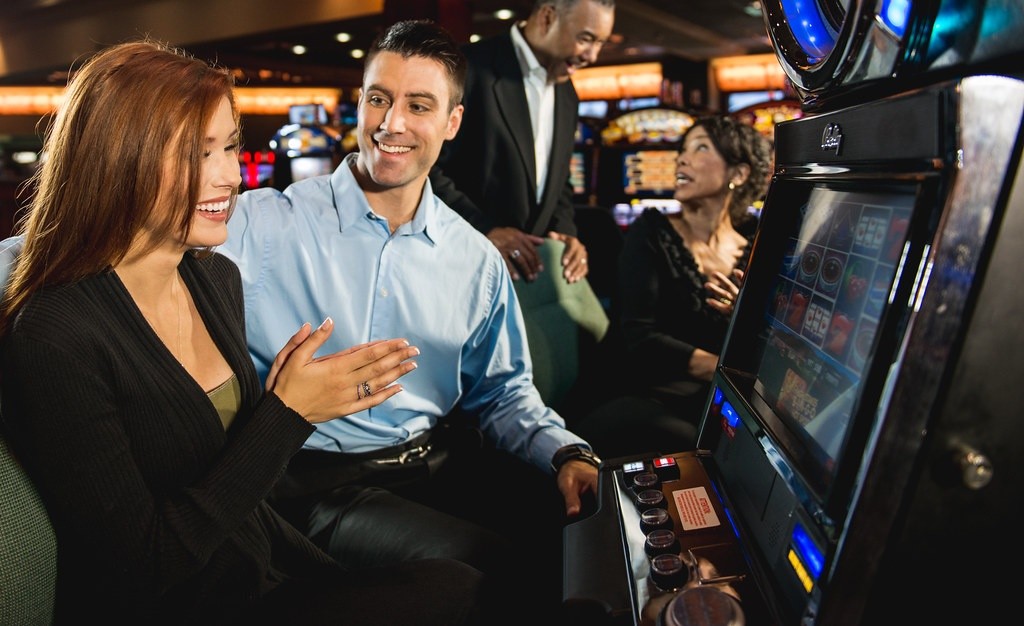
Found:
[0,230,610,626]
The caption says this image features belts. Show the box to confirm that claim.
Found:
[302,433,442,469]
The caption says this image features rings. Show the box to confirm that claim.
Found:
[581,258,586,264]
[431,0,615,281]
[512,250,520,258]
[362,382,371,396]
[357,385,361,400]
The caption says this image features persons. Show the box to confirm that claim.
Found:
[0,41,486,626]
[0,19,602,626]
[614,115,768,422]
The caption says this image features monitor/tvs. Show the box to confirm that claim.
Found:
[721,170,918,503]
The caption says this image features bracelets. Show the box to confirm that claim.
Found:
[550,446,602,483]
